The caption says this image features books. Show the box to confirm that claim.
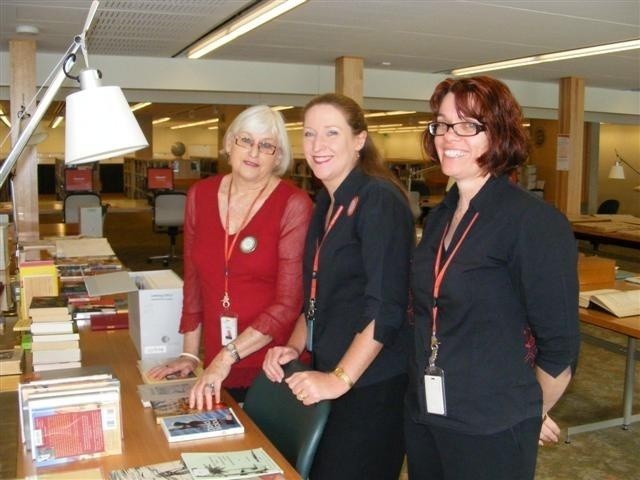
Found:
[0,219,285,480]
[568,211,639,238]
[578,287,640,319]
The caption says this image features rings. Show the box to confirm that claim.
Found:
[201,381,215,394]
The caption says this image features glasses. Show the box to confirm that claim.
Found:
[234,135,276,156]
[427,121,488,137]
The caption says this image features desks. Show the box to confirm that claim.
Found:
[572,210,640,430]
[1,291,308,480]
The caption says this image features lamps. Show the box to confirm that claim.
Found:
[607,149,639,181]
[0,0,151,190]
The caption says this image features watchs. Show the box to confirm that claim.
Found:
[222,340,244,365]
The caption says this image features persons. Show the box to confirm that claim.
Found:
[145,102,314,410]
[403,75,582,478]
[261,96,414,480]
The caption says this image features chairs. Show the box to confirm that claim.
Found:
[146,192,187,267]
[61,192,109,224]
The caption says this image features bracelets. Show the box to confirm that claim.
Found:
[322,362,357,392]
[177,347,206,363]
[541,409,548,424]
